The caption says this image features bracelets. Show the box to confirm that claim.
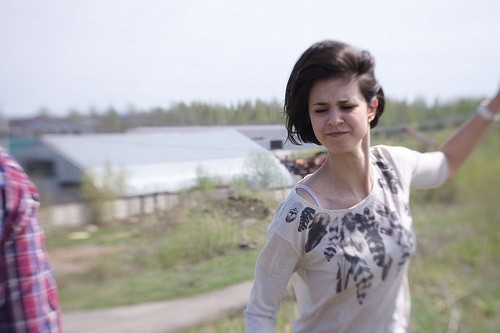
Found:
[476,100,498,122]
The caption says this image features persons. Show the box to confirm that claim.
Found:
[245,40,499,333]
[0,143,64,333]
